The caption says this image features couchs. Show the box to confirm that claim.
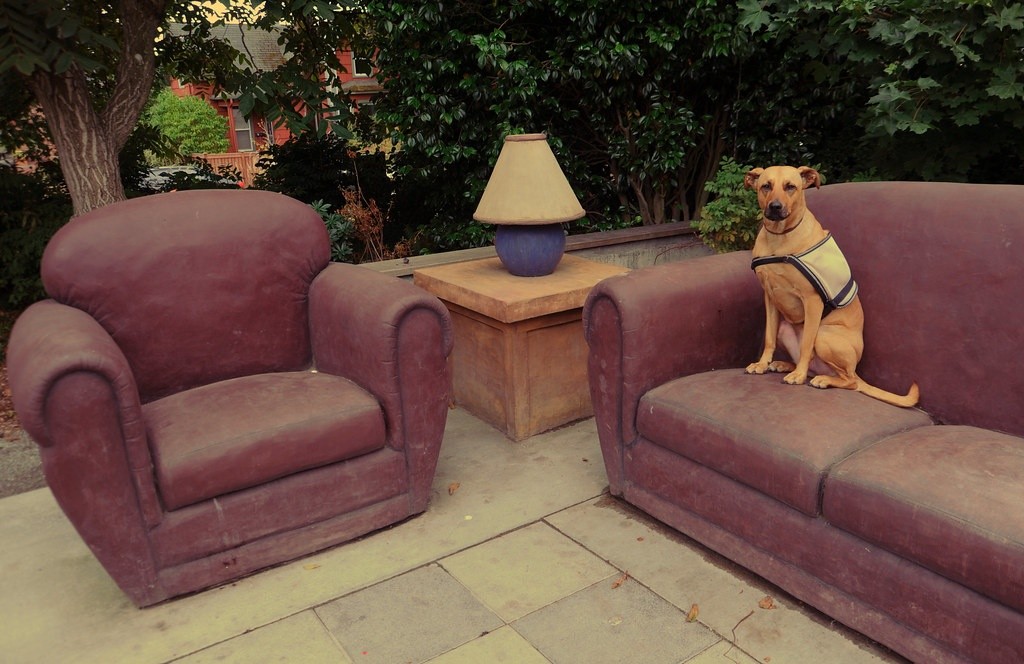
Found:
[5,188,457,614]
[578,178,1024,664]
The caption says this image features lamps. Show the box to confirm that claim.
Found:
[475,134,590,277]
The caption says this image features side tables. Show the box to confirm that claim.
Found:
[410,249,634,444]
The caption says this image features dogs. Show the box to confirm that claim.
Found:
[743,165,920,408]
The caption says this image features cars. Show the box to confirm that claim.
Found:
[141,166,246,193]
